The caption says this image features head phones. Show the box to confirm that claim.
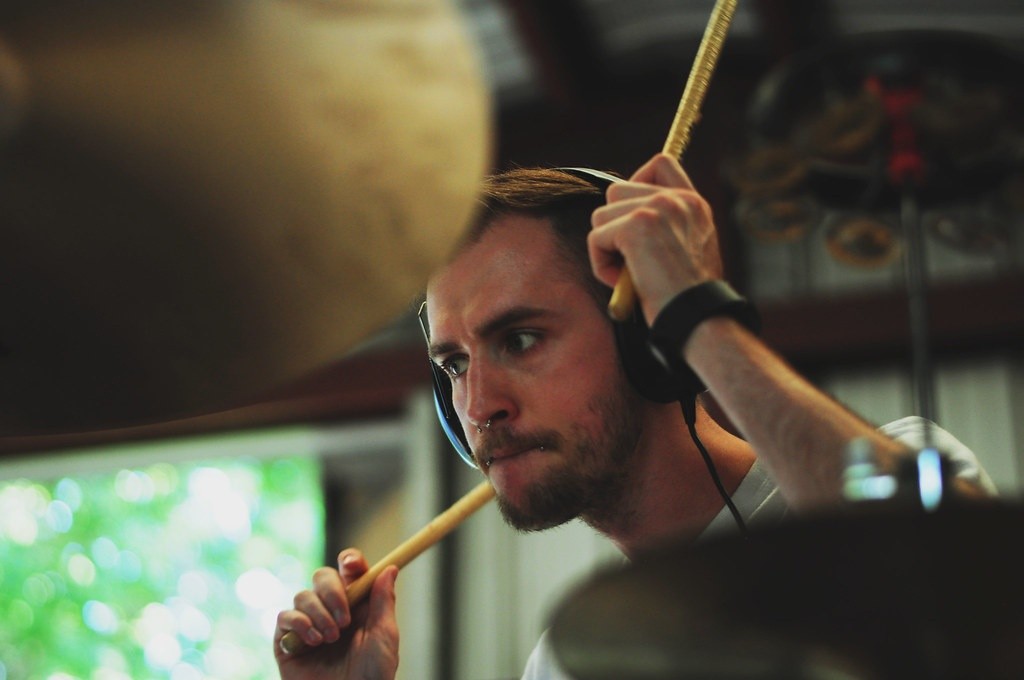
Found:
[420,167,710,468]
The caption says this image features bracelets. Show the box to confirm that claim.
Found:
[651,276,764,394]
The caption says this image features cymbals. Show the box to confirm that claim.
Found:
[549,496,1023,679]
[0,0,494,436]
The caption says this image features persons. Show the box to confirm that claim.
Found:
[276,154,1000,680]
